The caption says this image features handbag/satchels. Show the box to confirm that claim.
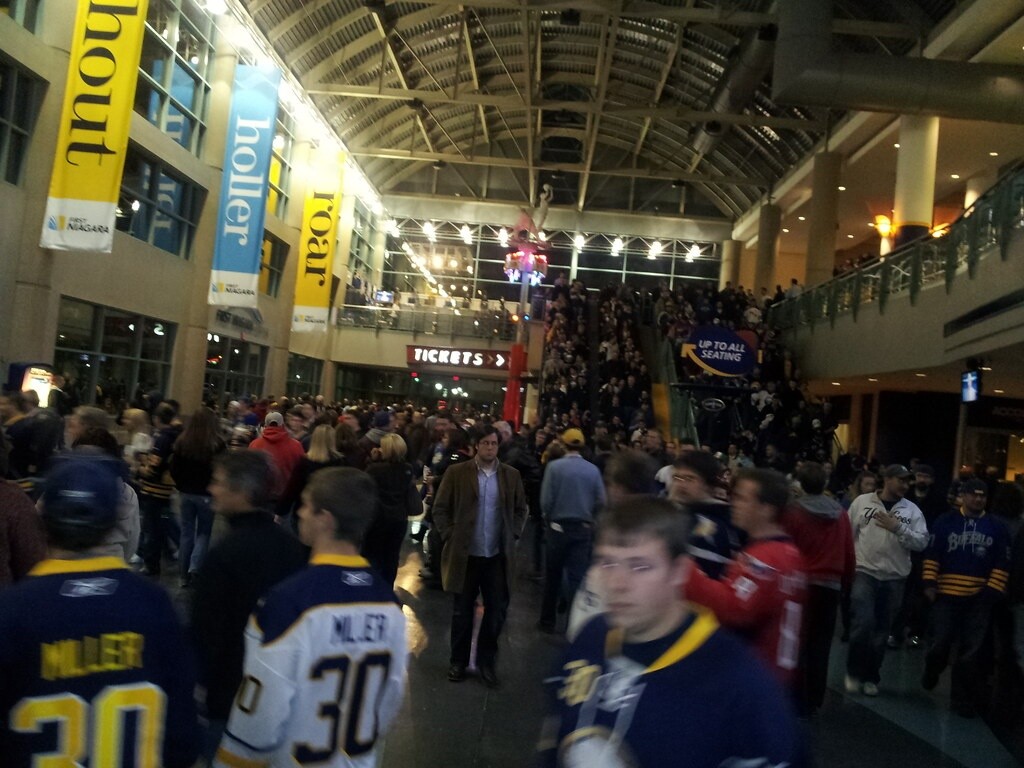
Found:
[406,483,424,516]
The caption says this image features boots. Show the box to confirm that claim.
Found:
[409,525,428,542]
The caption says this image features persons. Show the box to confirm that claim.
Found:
[843,464,930,695]
[537,187,553,231]
[432,424,527,689]
[782,463,855,719]
[0,252,1024,768]
[674,452,748,580]
[169,407,227,587]
[212,467,405,768]
[246,412,305,500]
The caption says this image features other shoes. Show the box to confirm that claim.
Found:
[845,675,860,693]
[537,620,554,634]
[448,665,467,681]
[907,635,923,648]
[864,681,879,696]
[419,567,433,577]
[842,629,850,641]
[178,576,187,589]
[922,666,936,690]
[138,564,163,576]
[478,666,500,688]
[887,635,901,650]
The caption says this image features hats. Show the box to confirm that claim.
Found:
[43,461,117,529]
[964,478,988,495]
[885,464,916,481]
[372,410,389,426]
[265,412,284,426]
[230,401,241,409]
[562,427,585,446]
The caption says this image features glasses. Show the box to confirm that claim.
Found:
[479,442,498,446]
[673,475,699,484]
